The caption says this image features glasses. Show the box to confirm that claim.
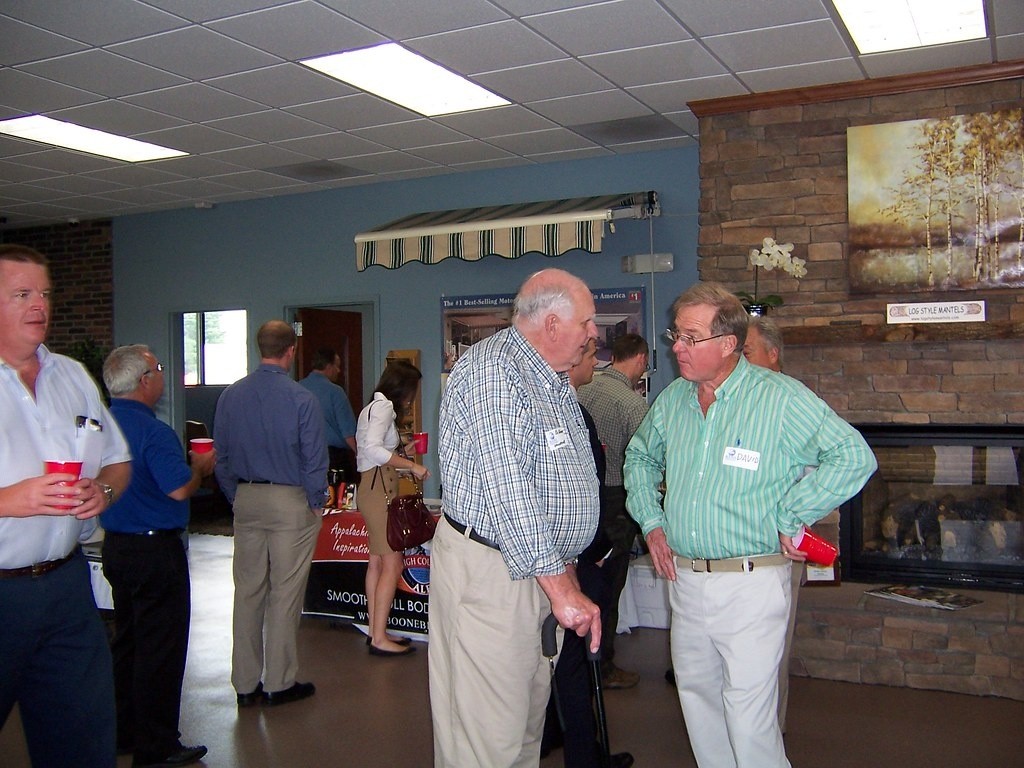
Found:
[138,363,164,384]
[665,328,737,350]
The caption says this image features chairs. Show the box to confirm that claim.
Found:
[186,420,223,520]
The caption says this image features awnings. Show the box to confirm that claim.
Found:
[354,193,658,272]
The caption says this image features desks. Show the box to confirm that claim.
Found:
[301,506,438,643]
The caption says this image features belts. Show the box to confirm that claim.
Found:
[445,512,500,549]
[672,550,792,572]
[0,542,81,579]
[139,527,180,537]
[237,476,272,484]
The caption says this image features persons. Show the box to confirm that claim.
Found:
[540,334,634,768]
[624,281,879,768]
[214,321,331,710]
[566,316,805,740]
[295,348,362,511]
[427,267,602,768]
[0,245,131,768]
[355,361,432,656]
[95,344,218,768]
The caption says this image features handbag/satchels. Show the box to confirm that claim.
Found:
[387,493,437,551]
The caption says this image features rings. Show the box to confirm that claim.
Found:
[782,550,788,556]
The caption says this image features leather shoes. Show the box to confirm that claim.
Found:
[260,674,315,705]
[132,743,207,768]
[608,751,634,768]
[540,733,564,760]
[236,680,264,707]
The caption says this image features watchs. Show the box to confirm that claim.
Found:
[99,483,113,506]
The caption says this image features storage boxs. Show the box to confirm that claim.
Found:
[628,553,672,629]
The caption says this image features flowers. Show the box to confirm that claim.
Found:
[731,237,808,307]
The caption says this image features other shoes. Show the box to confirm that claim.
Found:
[665,669,677,687]
[600,667,639,689]
[367,632,411,648]
[369,638,417,656]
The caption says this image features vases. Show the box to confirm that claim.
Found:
[743,306,768,318]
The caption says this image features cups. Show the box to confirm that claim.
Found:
[792,525,838,567]
[44,460,84,510]
[190,438,214,455]
[414,432,428,455]
